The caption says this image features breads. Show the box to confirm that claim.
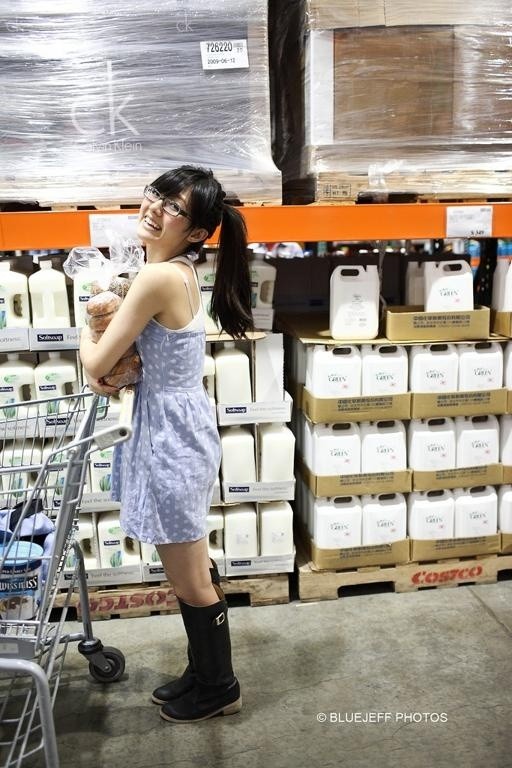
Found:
[85,277,141,390]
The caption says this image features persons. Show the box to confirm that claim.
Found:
[78,165,252,724]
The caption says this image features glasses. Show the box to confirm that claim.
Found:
[143,183,198,225]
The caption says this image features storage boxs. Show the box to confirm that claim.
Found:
[1,303,512,593]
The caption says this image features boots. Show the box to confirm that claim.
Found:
[162,584,242,724]
[151,555,221,705]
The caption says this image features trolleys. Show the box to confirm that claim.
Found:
[0,382,137,768]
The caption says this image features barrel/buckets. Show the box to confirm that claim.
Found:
[0,251,510,570]
